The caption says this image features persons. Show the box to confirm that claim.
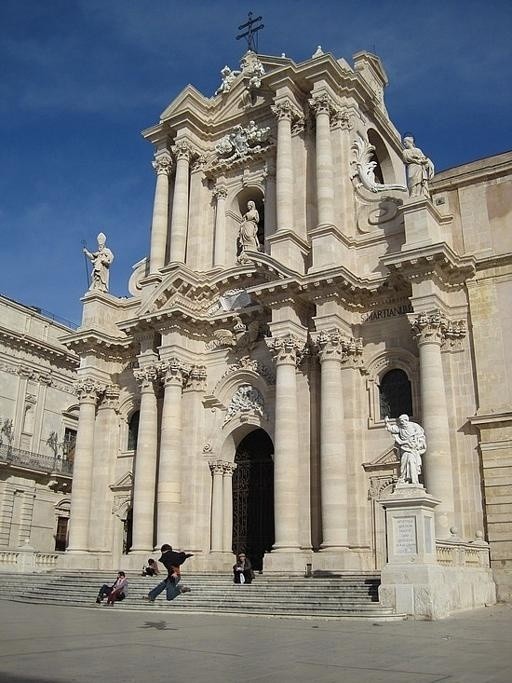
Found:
[239,200,260,247]
[384,414,427,484]
[96,571,128,606]
[233,553,255,584]
[141,559,160,577]
[83,241,114,293]
[143,544,194,601]
[402,137,435,199]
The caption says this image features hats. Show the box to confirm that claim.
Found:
[239,553,245,557]
